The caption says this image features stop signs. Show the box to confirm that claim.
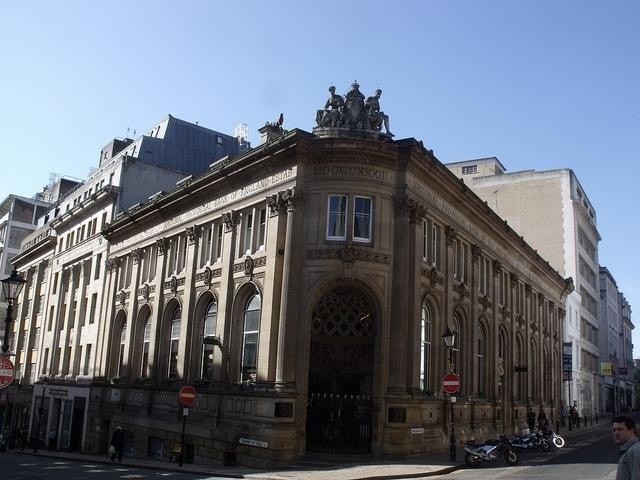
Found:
[443,374,461,394]
[0,356,15,389]
[177,385,196,406]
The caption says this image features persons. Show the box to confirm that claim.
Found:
[611,416,640,480]
[344,82,365,125]
[110,425,126,461]
[365,88,395,138]
[526,408,536,430]
[48,426,56,453]
[315,86,345,128]
[538,409,548,427]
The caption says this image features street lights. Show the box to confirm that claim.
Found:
[32,376,49,452]
[1,264,26,400]
[443,326,459,460]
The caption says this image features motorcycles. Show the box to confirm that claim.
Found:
[464,422,567,468]
